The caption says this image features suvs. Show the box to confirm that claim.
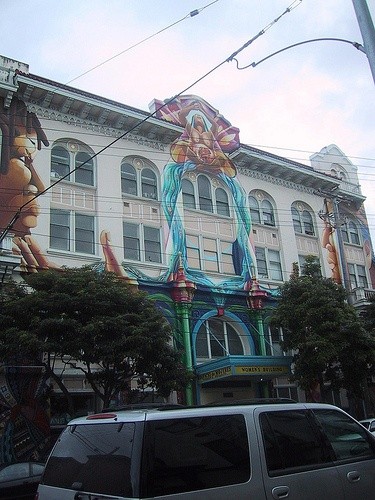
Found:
[34,397,375,500]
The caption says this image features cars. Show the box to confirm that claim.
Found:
[358,417,375,438]
